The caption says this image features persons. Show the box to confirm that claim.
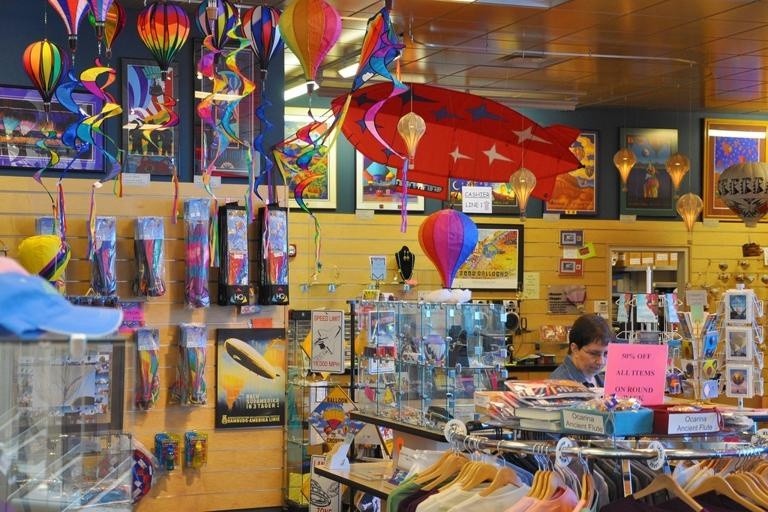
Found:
[547,313,612,390]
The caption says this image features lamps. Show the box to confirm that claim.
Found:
[336,57,360,79]
[509,23,536,222]
[614,59,636,191]
[398,12,426,170]
[284,78,321,102]
[676,69,704,244]
[666,70,690,200]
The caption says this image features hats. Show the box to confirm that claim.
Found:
[0,257,124,341]
[17,235,71,286]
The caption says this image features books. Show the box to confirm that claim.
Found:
[615,289,723,399]
[472,378,599,431]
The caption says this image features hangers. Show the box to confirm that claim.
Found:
[479,439,524,497]
[634,447,768,512]
[526,442,593,512]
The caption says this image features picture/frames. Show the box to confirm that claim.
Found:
[619,126,680,217]
[702,118,768,222]
[542,129,600,216]
[725,291,753,399]
[442,178,521,215]
[121,57,181,176]
[449,223,525,292]
[356,149,425,211]
[278,106,337,209]
[192,37,264,178]
[0,84,107,173]
[0,336,125,437]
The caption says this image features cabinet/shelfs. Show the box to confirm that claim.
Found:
[281,301,349,512]
[350,300,507,434]
[314,410,507,511]
